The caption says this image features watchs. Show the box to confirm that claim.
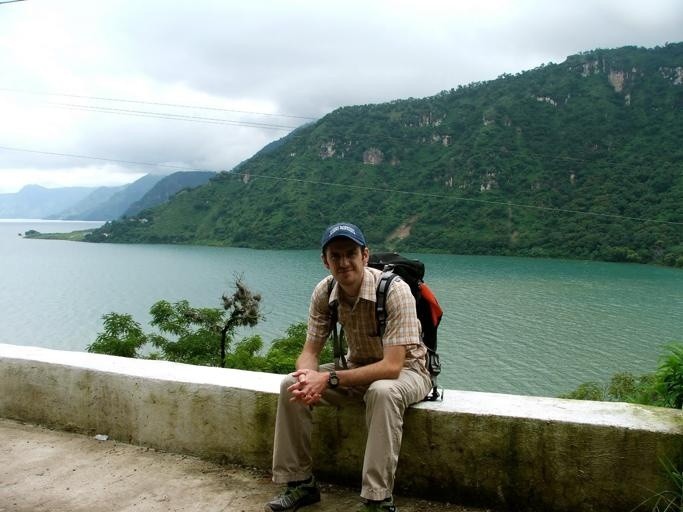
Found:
[328,371,338,387]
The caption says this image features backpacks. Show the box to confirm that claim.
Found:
[328,252,443,375]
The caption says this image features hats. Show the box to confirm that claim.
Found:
[321,222,366,249]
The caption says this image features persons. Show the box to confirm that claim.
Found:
[264,222,433,512]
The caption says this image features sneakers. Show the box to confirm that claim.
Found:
[264,475,320,512]
[353,496,396,512]
